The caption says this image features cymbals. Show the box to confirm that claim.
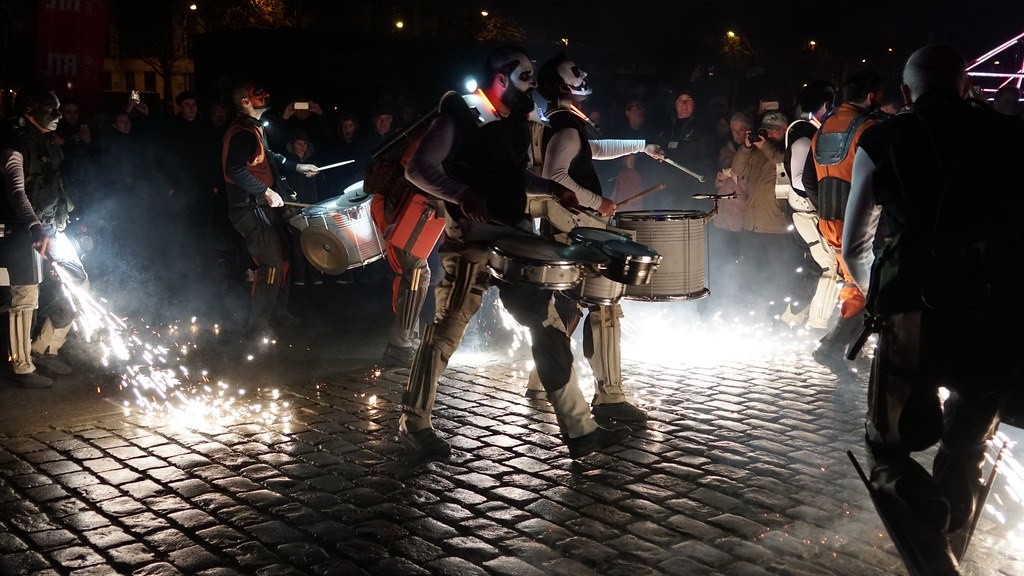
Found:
[554,242,609,263]
[300,223,351,276]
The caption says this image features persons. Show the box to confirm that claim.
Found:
[393,42,633,462]
[842,42,1023,575]
[533,53,667,426]
[0,87,74,392]
[0,35,1024,377]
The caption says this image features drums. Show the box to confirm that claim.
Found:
[615,209,711,304]
[565,226,661,307]
[484,234,587,288]
[299,185,387,272]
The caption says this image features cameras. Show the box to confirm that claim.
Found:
[748,129,767,143]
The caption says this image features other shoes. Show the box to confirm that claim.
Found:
[567,427,632,457]
[32,351,72,376]
[248,323,279,343]
[18,371,51,386]
[273,311,299,324]
[592,400,647,421]
[400,430,451,456]
[384,345,417,367]
[846,354,874,371]
[814,347,856,383]
[526,388,550,400]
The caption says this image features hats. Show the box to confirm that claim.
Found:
[759,112,790,132]
